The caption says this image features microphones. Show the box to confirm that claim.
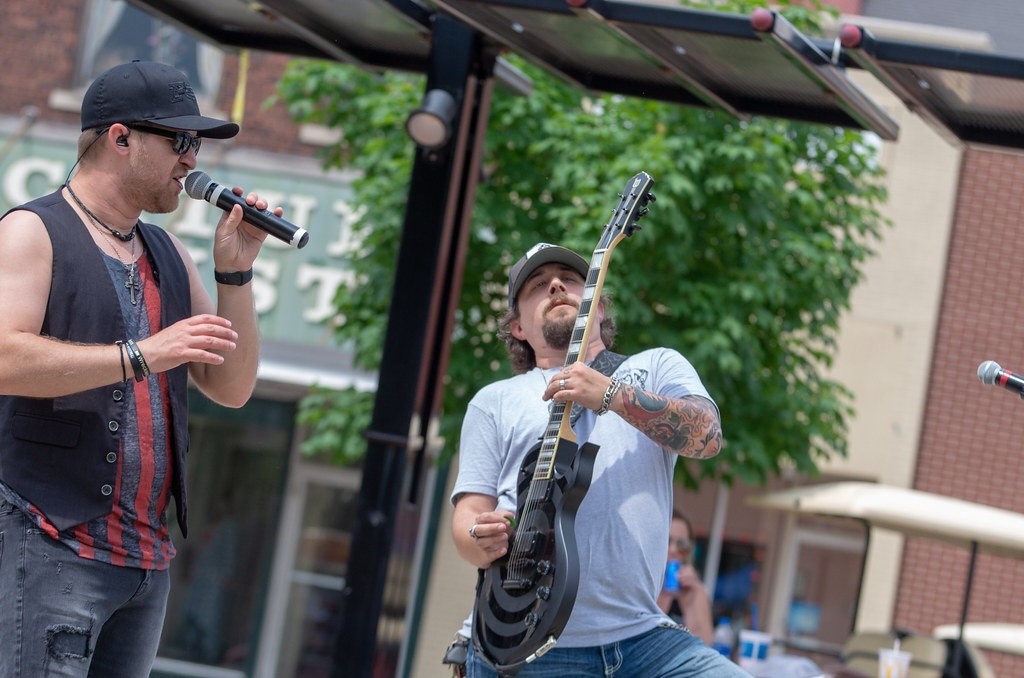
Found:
[184,171,310,250]
[976,360,1024,398]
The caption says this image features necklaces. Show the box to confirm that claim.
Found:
[66,181,140,305]
[541,359,595,413]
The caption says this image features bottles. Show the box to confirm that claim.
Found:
[713,617,735,661]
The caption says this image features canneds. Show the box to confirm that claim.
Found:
[664,560,681,592]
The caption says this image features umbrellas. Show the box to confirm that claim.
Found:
[746,480,1024,677]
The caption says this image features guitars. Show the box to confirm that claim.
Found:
[471,170,657,674]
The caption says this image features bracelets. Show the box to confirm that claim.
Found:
[115,338,150,383]
[214,269,252,286]
[593,377,621,416]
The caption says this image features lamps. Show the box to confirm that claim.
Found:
[404,14,472,147]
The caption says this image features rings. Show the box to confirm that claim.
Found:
[469,525,476,538]
[559,380,565,389]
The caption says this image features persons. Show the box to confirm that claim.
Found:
[656,509,713,644]
[0,59,283,678]
[442,242,754,678]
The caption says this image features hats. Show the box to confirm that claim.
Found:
[508,242,590,310]
[80,60,239,140]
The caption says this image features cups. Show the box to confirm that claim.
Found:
[878,646,912,678]
[739,628,772,670]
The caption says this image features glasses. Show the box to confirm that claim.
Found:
[94,123,201,156]
[668,536,692,556]
